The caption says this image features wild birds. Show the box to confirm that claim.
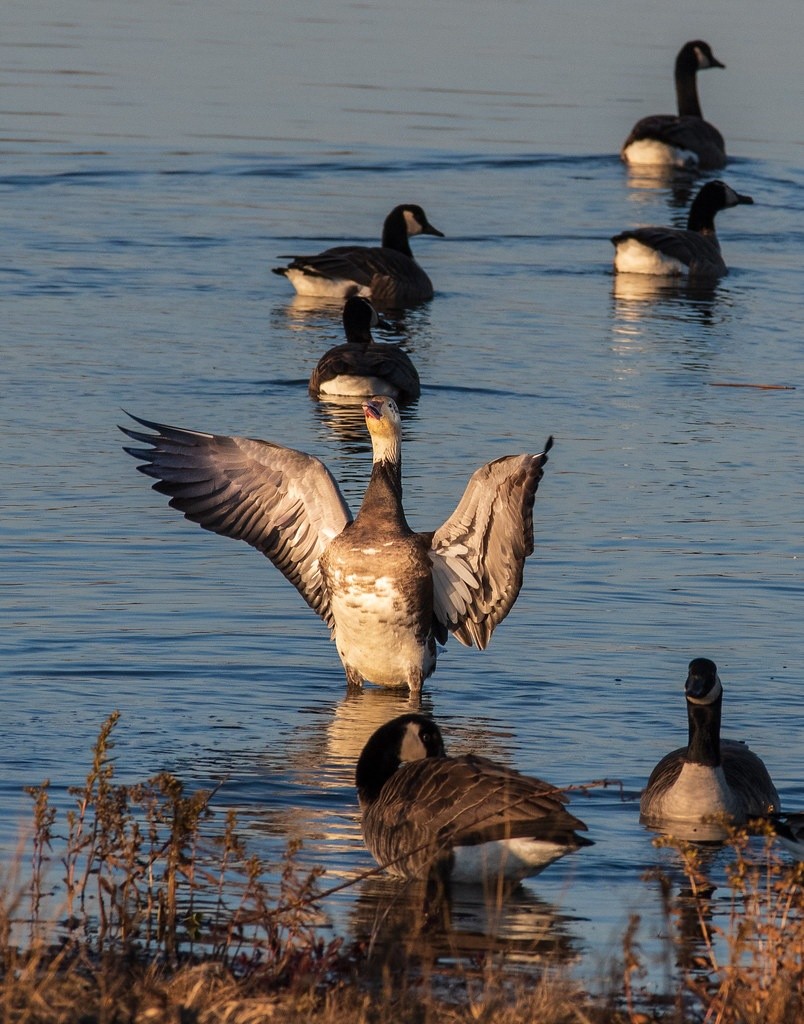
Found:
[611,180,754,276]
[271,204,446,310]
[113,396,554,699]
[308,296,420,396]
[620,40,727,170]
[356,714,596,884]
[640,657,781,822]
